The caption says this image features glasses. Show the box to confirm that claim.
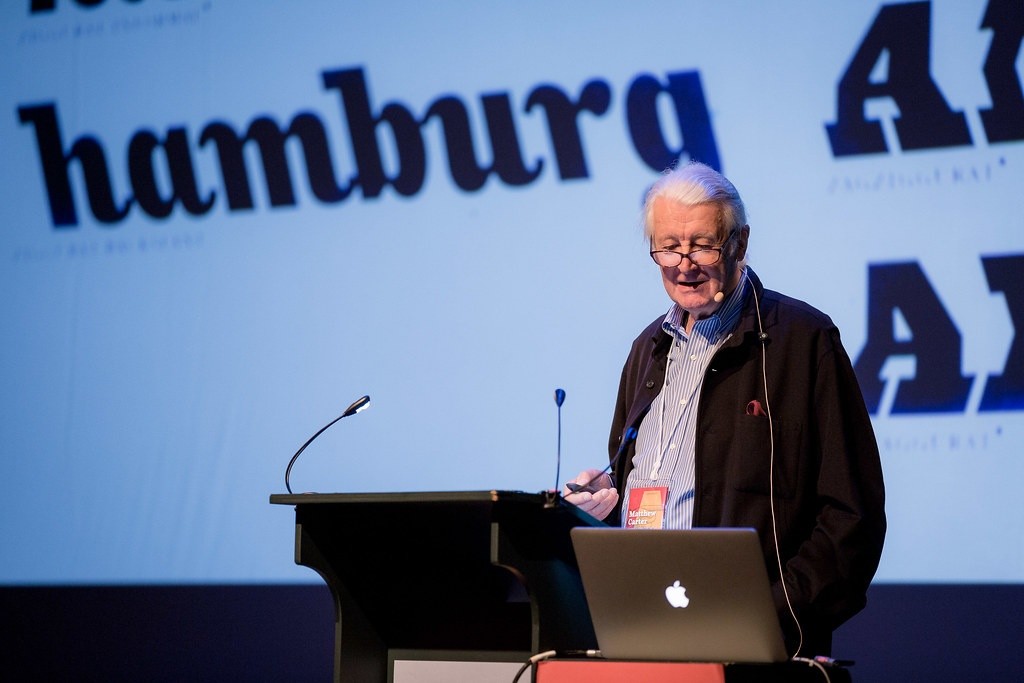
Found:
[649,228,739,268]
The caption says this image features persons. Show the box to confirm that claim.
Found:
[563,160,887,660]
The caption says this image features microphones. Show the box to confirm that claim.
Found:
[286,397,371,494]
[714,290,727,304]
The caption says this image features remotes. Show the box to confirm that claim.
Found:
[567,483,596,496]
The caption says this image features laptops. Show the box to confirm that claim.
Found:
[572,526,856,667]
